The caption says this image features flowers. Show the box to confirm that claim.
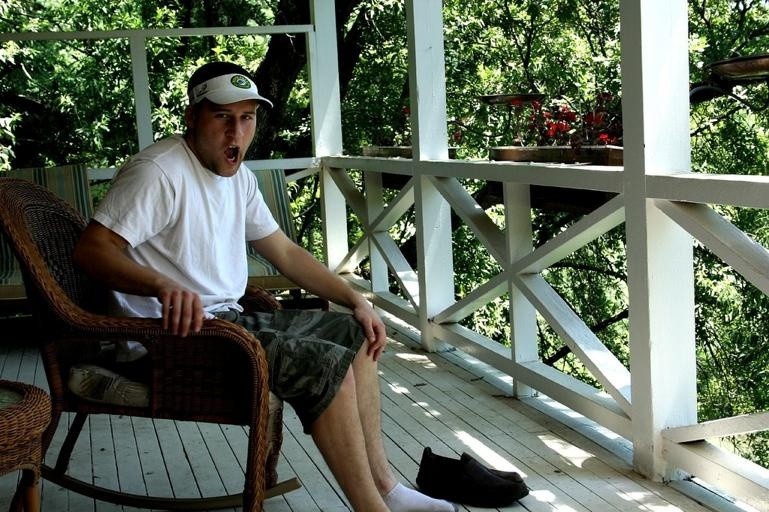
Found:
[505,90,621,146]
[392,106,412,147]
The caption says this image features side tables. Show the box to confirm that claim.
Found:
[1,379,52,511]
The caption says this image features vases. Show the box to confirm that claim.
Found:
[485,146,624,215]
[363,147,462,189]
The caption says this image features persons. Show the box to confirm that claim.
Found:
[72,62,453,512]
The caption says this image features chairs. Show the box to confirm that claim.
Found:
[0,162,94,318]
[0,178,332,511]
[246,169,302,300]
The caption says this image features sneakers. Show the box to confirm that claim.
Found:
[416,446,528,508]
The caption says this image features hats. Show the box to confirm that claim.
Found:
[187,72,275,108]
